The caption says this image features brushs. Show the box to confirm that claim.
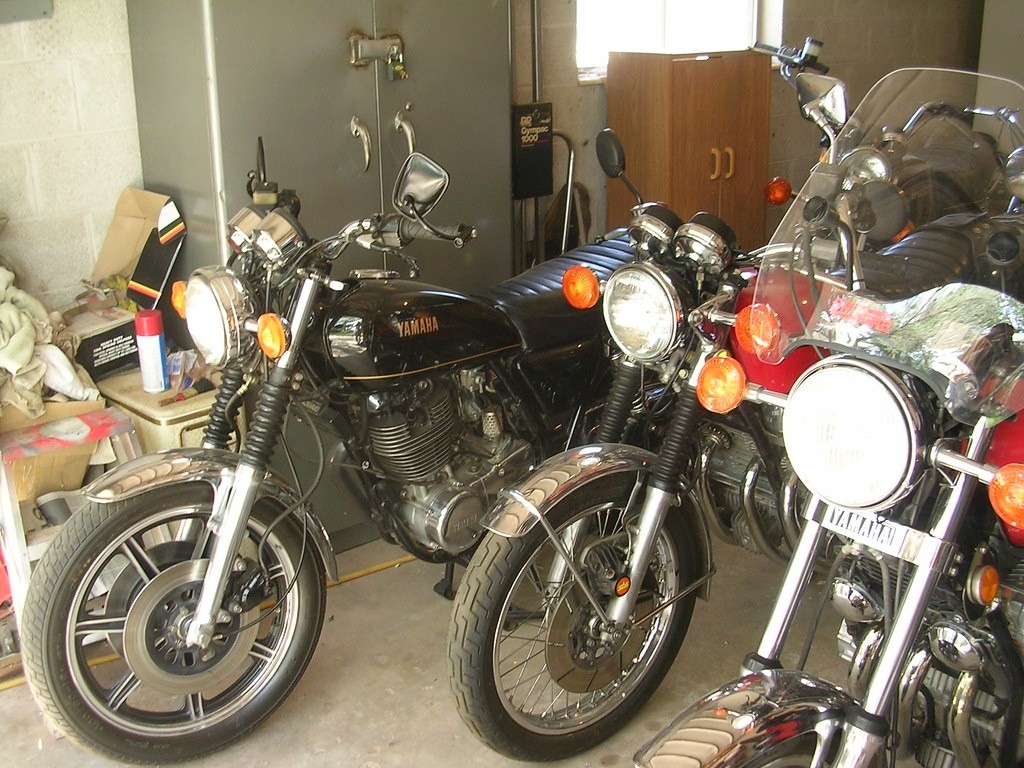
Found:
[158,377,216,408]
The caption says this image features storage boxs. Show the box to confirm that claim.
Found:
[43,187,188,384]
[0,392,106,505]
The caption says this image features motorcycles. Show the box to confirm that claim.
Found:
[16,136,707,768]
[633,35,1023,768]
[442,128,1024,760]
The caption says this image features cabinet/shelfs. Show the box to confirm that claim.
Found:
[96,357,259,582]
[127,0,511,560]
[606,51,772,256]
